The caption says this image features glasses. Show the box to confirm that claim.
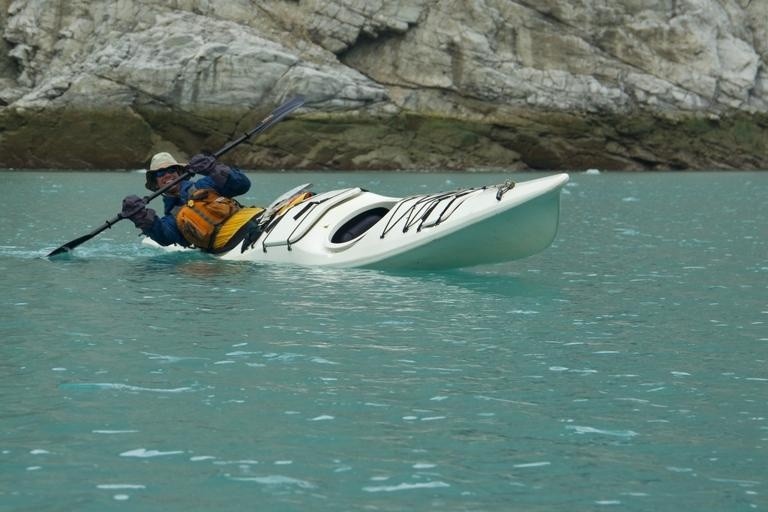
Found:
[154,169,180,178]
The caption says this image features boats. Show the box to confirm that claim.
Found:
[142,172,569,271]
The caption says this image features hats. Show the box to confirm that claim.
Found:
[145,151,187,190]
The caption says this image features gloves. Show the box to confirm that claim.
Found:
[121,195,155,228]
[190,154,229,187]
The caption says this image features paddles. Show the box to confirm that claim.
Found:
[47,93,309,256]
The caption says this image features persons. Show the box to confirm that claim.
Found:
[121,151,262,253]
